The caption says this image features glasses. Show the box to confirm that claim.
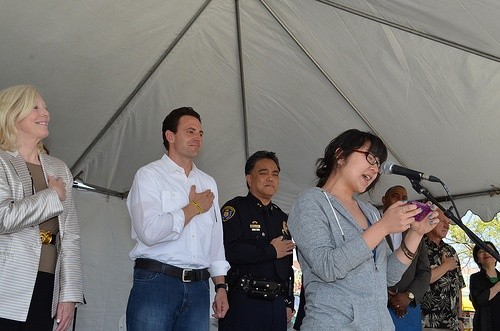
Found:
[351,149,381,170]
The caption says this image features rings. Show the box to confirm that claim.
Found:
[397,305,399,307]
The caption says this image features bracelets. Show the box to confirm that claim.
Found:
[190,201,202,214]
[401,238,418,260]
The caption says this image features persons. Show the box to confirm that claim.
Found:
[218,150,296,331]
[469,241,500,331]
[286,128,441,330]
[0,83,85,331]
[125,106,231,331]
[379,184,473,331]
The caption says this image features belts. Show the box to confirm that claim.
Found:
[134,258,211,283]
[40,231,57,246]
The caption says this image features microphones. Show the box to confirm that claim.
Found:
[380,161,441,182]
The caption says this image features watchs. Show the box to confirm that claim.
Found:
[405,291,415,300]
[458,317,465,323]
[215,283,229,292]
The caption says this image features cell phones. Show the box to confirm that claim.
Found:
[406,200,432,222]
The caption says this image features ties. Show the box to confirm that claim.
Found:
[261,205,282,266]
